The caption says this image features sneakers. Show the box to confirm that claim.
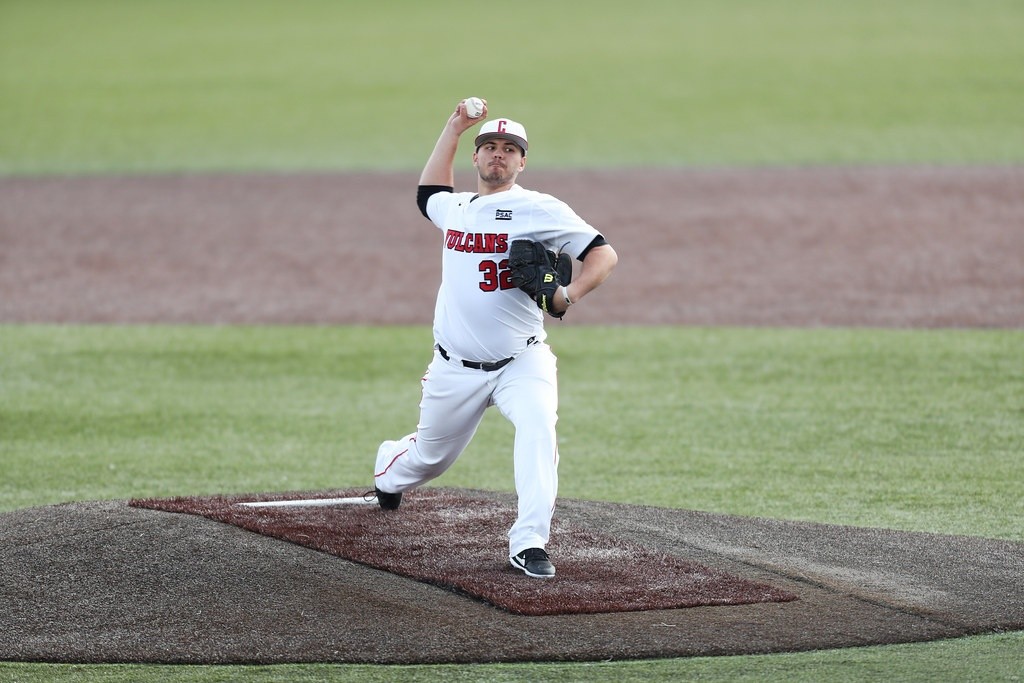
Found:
[510,547,556,580]
[363,485,402,510]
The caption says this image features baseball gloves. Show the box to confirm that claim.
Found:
[508,239,573,319]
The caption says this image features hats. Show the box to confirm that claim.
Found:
[475,118,529,153]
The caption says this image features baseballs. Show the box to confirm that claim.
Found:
[464,97,485,119]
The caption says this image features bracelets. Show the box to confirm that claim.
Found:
[562,286,575,307]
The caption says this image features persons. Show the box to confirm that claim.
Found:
[375,96,619,578]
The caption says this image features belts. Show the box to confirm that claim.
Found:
[438,335,538,372]
